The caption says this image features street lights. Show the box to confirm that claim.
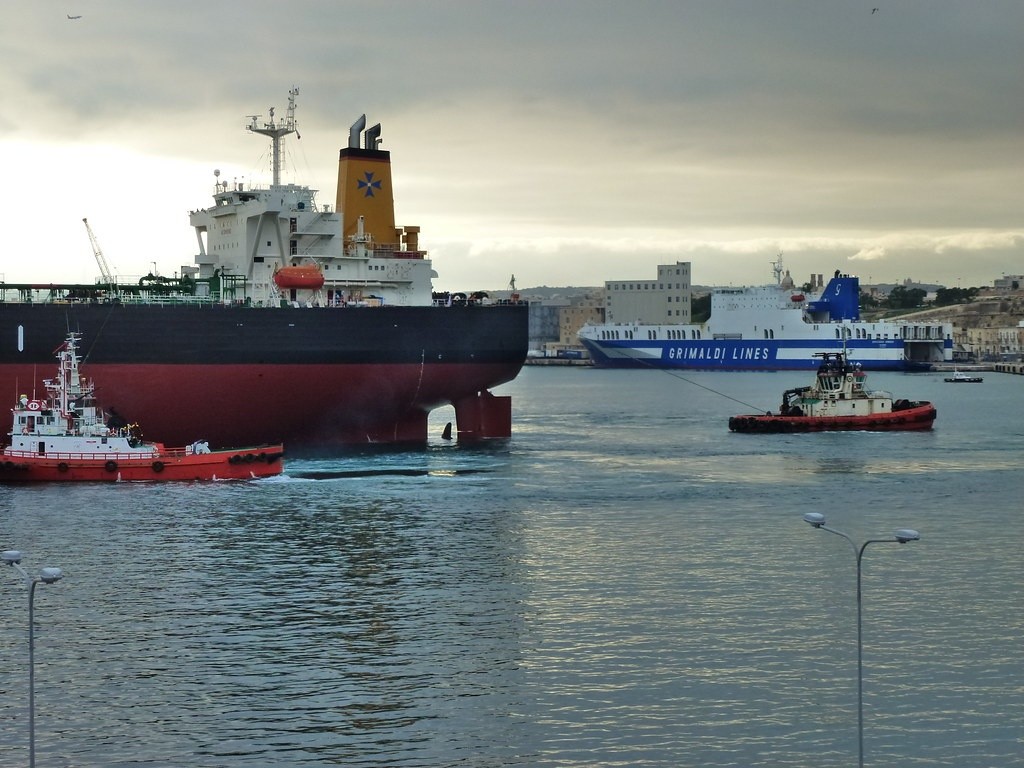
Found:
[1,549,67,767]
[804,511,922,767]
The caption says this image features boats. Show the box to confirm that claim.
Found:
[0,312,283,482]
[0,85,528,447]
[575,248,905,370]
[276,267,324,290]
[944,363,983,382]
[727,323,937,431]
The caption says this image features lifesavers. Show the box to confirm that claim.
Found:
[22,427,29,435]
